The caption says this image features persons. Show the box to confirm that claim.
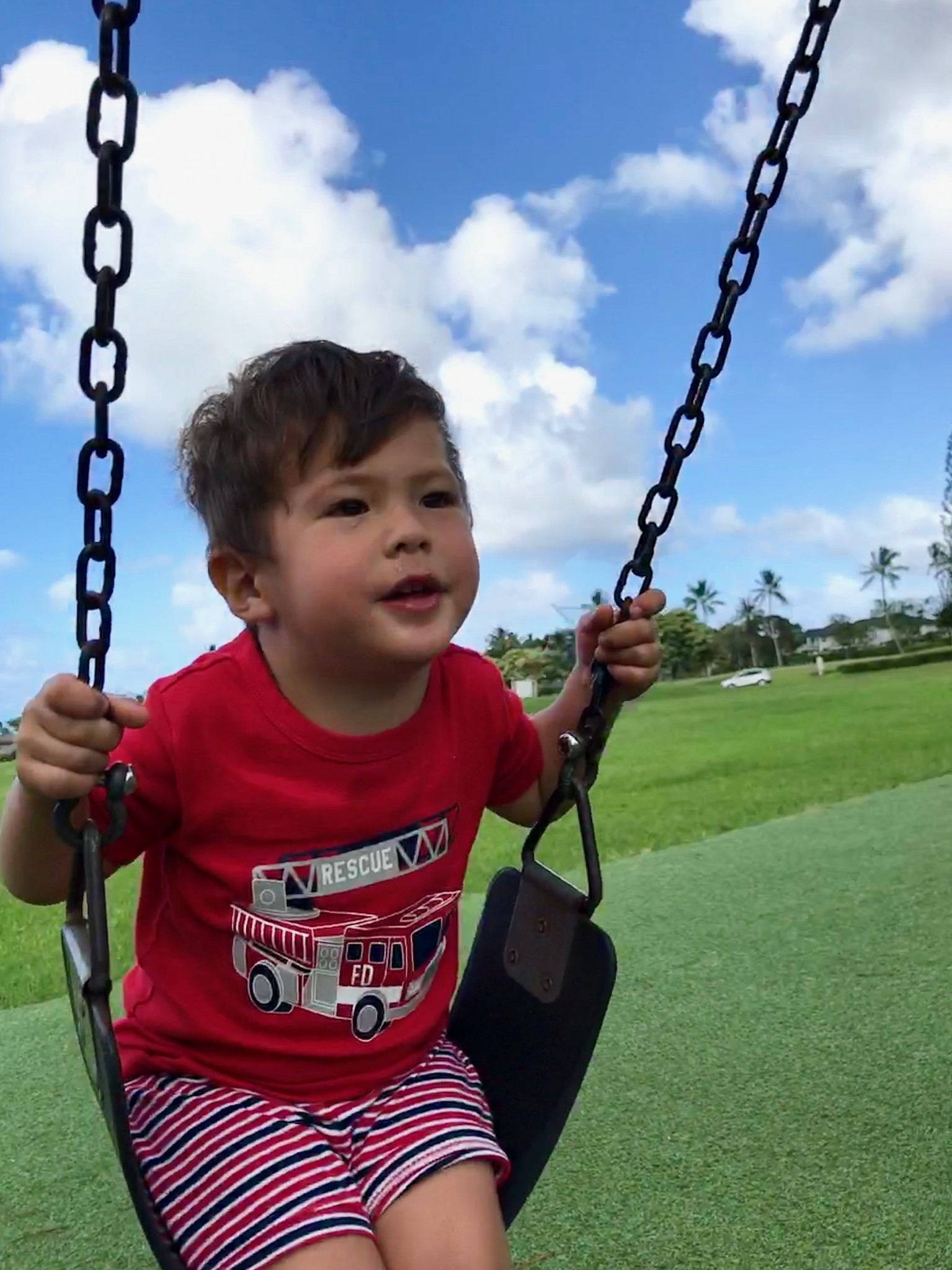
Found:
[0,338,670,1269]
[816,654,825,676]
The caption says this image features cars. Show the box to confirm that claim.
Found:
[720,667,771,689]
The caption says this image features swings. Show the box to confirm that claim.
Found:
[56,0,848,1270]
